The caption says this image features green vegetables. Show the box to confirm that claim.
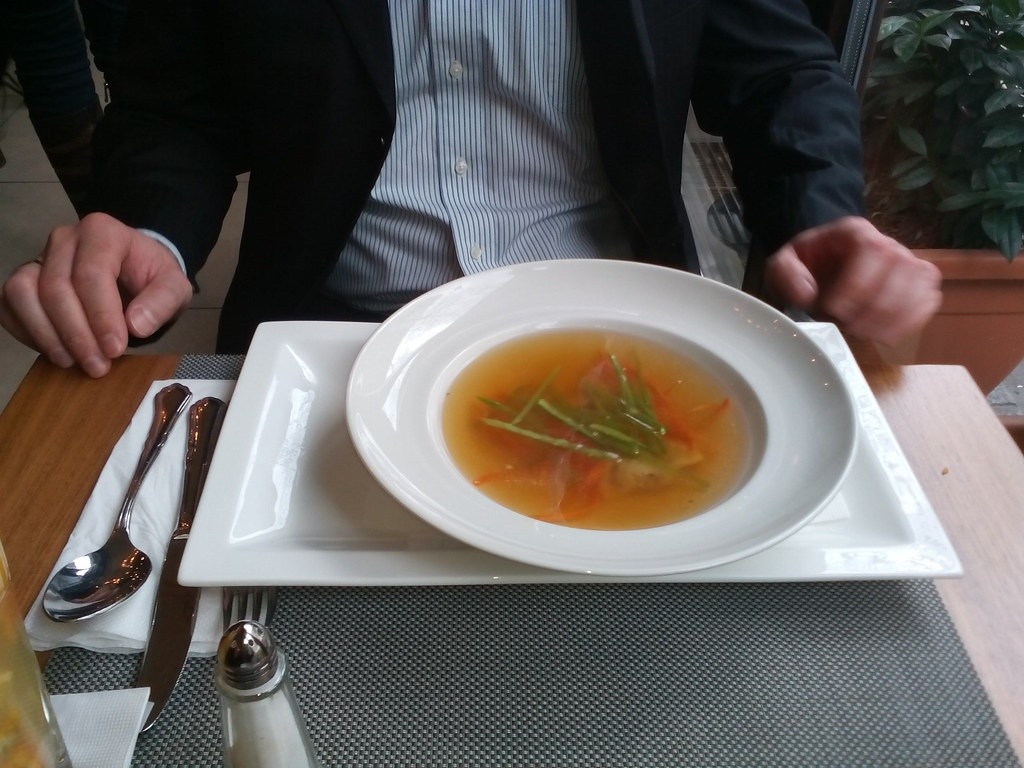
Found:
[476,351,672,467]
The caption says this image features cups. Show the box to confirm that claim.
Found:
[0,542,74,768]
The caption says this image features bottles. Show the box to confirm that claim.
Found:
[215,621,320,768]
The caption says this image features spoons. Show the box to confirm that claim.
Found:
[43,383,192,622]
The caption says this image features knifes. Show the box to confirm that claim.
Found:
[137,397,229,733]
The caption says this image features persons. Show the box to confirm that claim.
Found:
[0,0,946,379]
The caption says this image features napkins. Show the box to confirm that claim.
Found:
[13,376,268,657]
[46,684,153,768]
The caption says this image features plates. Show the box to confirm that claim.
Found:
[178,321,964,586]
[344,260,858,578]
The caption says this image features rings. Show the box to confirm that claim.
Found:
[21,259,44,269]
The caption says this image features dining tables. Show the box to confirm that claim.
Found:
[0,349,1024,768]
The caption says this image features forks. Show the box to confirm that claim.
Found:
[221,586,276,634]
